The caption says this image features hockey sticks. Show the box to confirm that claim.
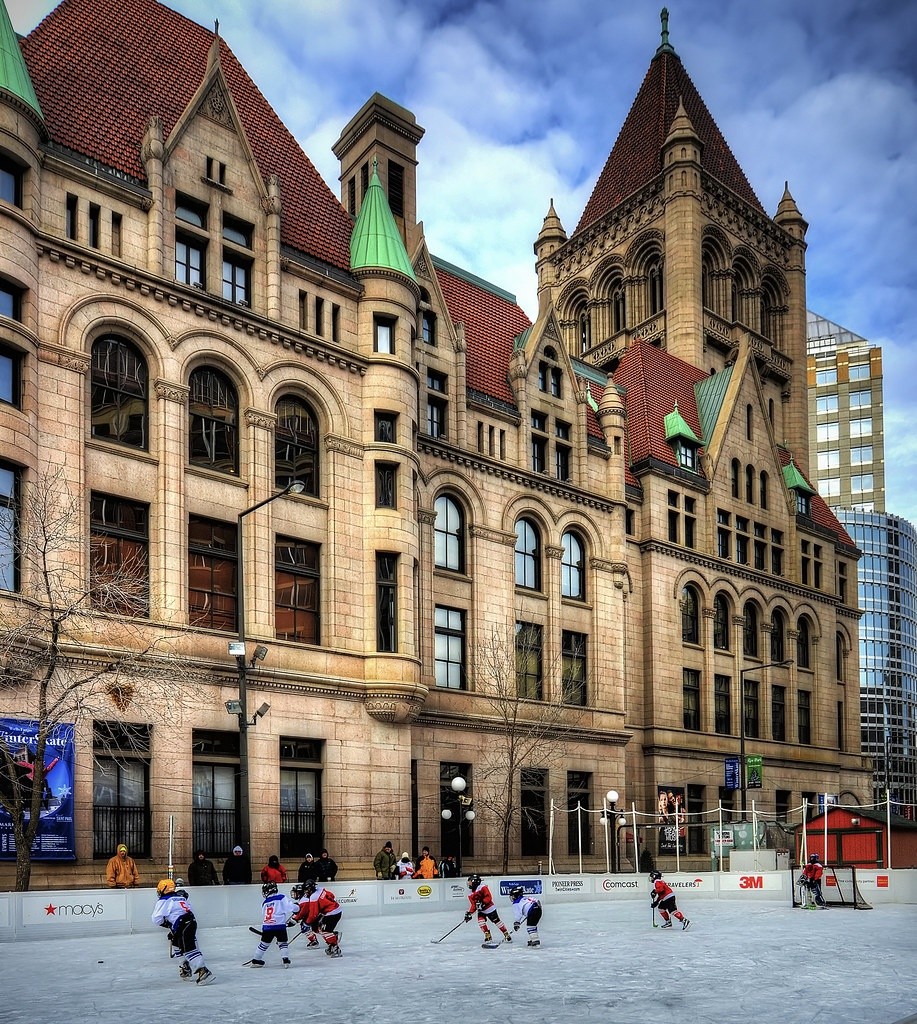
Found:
[249,913,294,936]
[480,916,527,949]
[241,958,254,966]
[653,893,658,927]
[169,940,175,958]
[275,914,321,947]
[430,909,478,944]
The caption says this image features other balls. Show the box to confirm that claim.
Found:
[98,961,103,963]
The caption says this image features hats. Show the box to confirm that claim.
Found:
[320,849,328,859]
[382,841,430,859]
[306,853,313,858]
[233,845,242,855]
[120,847,126,852]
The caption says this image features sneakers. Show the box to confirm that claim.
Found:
[680,918,692,932]
[484,929,540,949]
[661,917,672,928]
[179,931,344,984]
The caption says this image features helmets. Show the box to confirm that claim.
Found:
[290,879,317,900]
[262,880,278,899]
[157,879,175,898]
[468,874,482,889]
[175,890,188,900]
[649,870,661,884]
[809,853,819,863]
[509,886,523,902]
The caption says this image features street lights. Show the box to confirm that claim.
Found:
[225,481,307,855]
[887,735,911,789]
[442,777,475,876]
[740,660,794,821]
[599,790,627,873]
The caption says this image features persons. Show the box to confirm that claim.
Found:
[649,869,692,931]
[412,846,439,879]
[297,852,318,883]
[251,880,300,964]
[17,755,60,810]
[464,875,511,941]
[315,848,338,882]
[151,878,213,984]
[437,854,459,878]
[286,879,343,957]
[260,855,288,883]
[223,845,253,884]
[106,844,139,889]
[510,886,543,946]
[374,841,397,880]
[658,790,686,834]
[396,851,415,879]
[188,849,221,886]
[799,852,826,906]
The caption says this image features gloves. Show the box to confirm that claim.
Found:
[288,918,298,927]
[159,919,172,928]
[651,901,659,909]
[476,901,485,911]
[651,889,656,898]
[301,921,310,933]
[514,921,520,931]
[167,931,174,941]
[465,911,472,923]
